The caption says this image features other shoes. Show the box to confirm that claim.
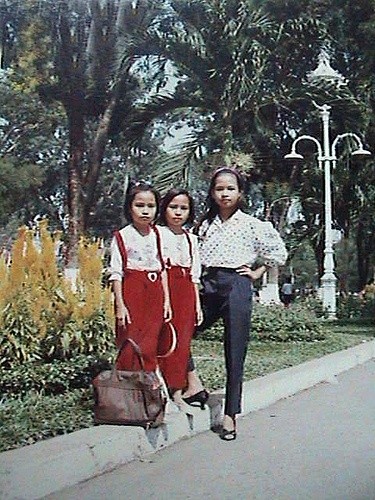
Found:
[163,401,180,415]
[174,399,193,413]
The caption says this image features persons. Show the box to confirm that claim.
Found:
[366,278,375,287]
[281,279,292,303]
[181,168,288,441]
[110,185,172,378]
[157,188,203,416]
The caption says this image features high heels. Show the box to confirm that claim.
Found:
[220,412,236,441]
[181,389,209,410]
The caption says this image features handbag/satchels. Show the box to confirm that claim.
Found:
[92,337,166,429]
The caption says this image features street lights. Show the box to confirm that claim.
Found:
[284,100,372,322]
